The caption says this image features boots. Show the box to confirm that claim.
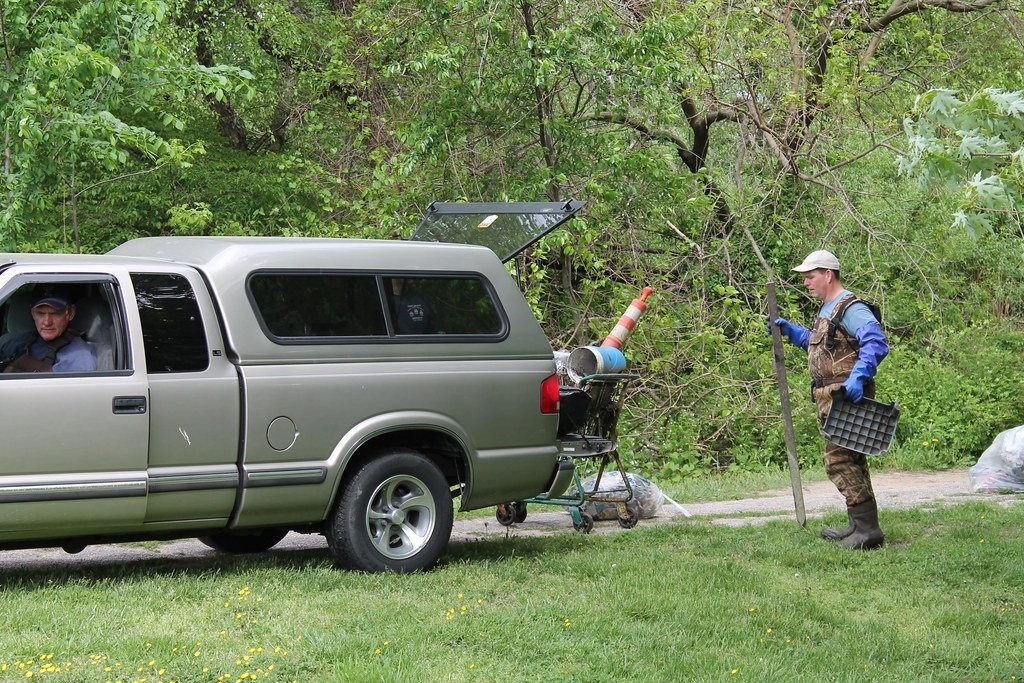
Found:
[821,510,856,540]
[834,499,884,548]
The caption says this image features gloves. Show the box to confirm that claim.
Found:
[842,323,889,404]
[765,314,812,352]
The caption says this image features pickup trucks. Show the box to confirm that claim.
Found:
[0,197,589,574]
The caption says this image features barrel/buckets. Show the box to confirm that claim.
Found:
[567,346,627,384]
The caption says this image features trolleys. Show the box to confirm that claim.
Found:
[494,373,640,531]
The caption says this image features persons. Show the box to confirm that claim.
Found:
[765,250,890,550]
[0,284,98,372]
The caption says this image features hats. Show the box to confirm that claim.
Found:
[29,283,76,310]
[790,250,840,272]
